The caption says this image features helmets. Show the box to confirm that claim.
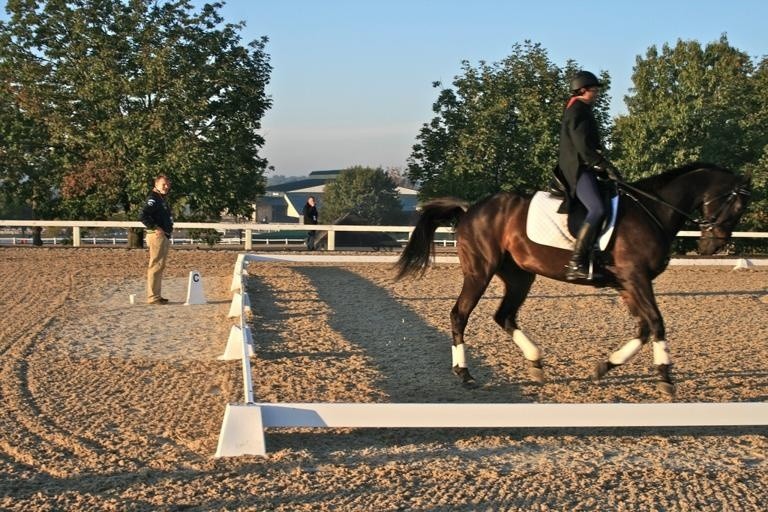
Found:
[569,70,608,91]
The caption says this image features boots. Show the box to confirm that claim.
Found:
[565,223,614,281]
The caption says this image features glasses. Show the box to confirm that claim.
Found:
[589,89,600,93]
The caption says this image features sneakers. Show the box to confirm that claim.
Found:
[149,298,167,305]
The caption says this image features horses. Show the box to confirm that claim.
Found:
[389,160,753,397]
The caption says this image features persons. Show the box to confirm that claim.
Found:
[303,196,319,251]
[140,174,172,305]
[559,72,611,283]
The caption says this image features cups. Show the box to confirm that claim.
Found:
[129,294,136,304]
